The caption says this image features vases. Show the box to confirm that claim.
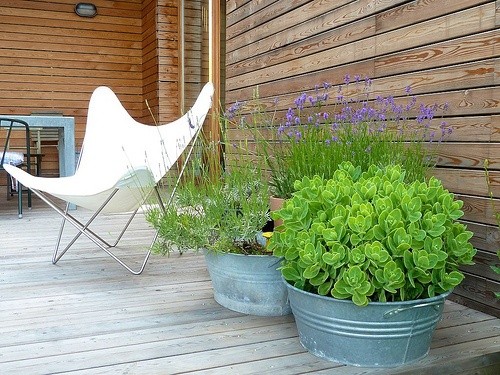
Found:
[269,193,286,233]
[200,245,293,318]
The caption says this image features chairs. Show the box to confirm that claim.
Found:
[2,81,218,276]
[1,117,34,219]
[2,126,46,201]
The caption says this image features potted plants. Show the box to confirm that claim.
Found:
[269,160,480,368]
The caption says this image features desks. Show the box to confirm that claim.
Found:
[0,114,78,211]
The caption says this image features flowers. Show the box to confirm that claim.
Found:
[268,71,456,201]
[222,93,304,197]
[117,84,275,257]
